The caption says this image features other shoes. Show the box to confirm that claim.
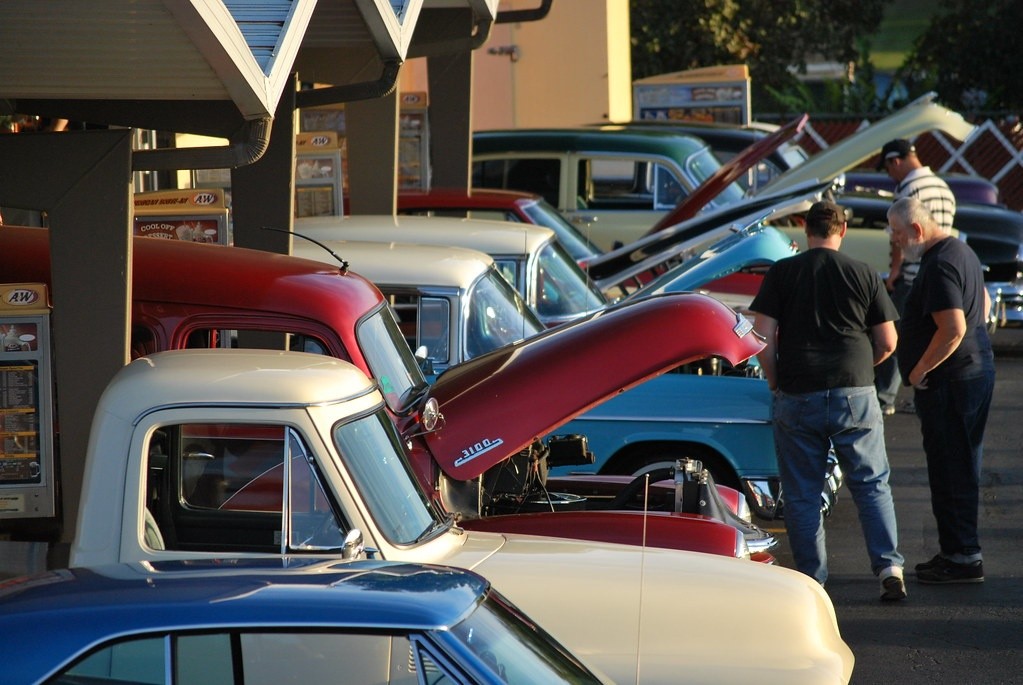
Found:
[880,565,907,602]
[915,552,984,583]
[881,404,895,414]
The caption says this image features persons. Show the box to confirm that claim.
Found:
[748,201,907,604]
[887,196,995,582]
[872,139,957,415]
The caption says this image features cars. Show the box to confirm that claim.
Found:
[0,90,1023,685]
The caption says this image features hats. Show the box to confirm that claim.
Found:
[806,199,846,224]
[876,138,915,171]
